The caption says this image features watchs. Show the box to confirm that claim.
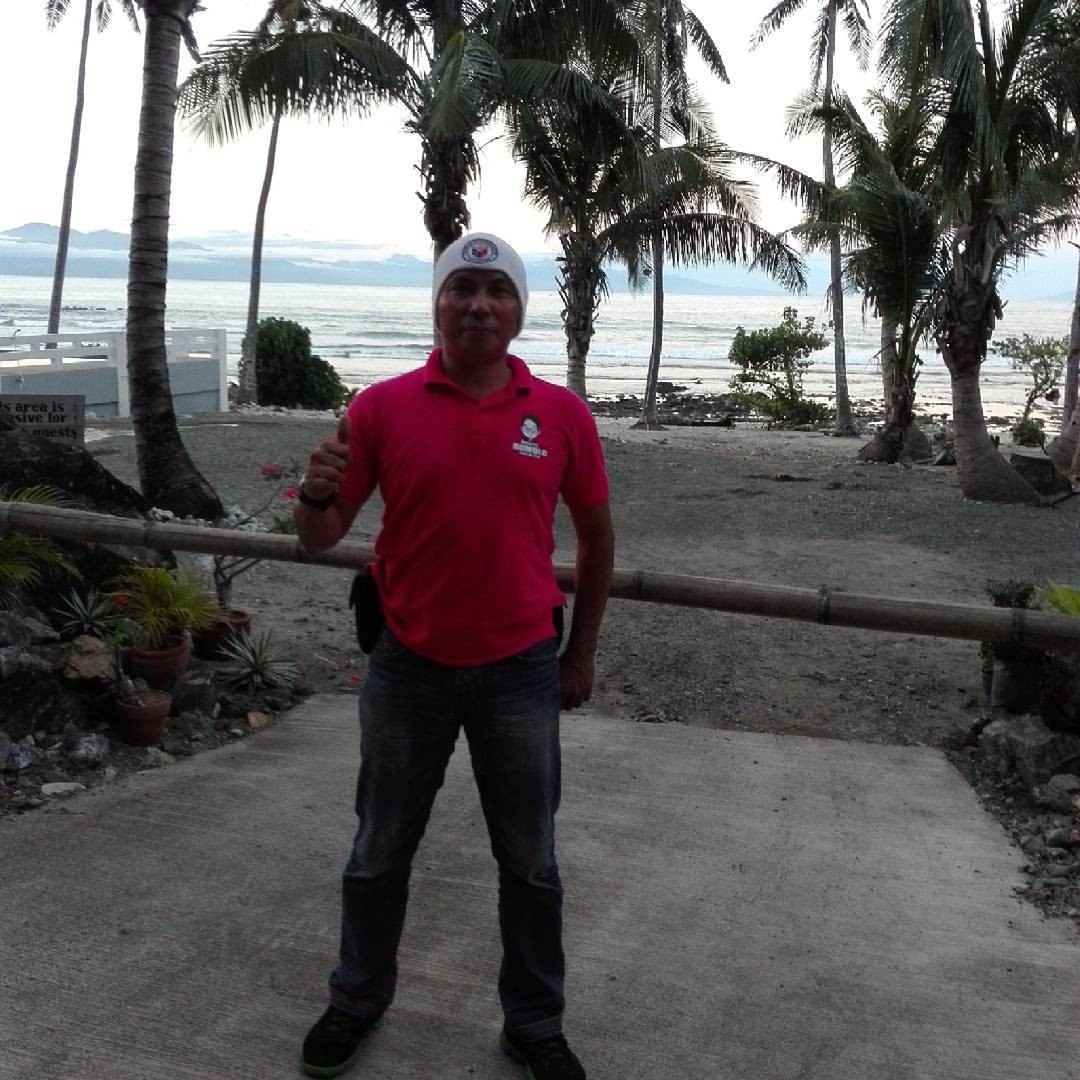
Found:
[298,474,336,511]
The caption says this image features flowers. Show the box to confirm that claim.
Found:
[214,459,298,610]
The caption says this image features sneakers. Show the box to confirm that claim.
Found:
[501,1024,585,1080]
[302,1004,384,1074]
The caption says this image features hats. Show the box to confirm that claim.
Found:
[431,232,527,339]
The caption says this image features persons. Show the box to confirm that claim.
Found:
[293,233,616,1080]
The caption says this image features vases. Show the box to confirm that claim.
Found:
[192,610,251,661]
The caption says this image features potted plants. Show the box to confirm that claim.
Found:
[116,687,171,747]
[121,561,207,685]
